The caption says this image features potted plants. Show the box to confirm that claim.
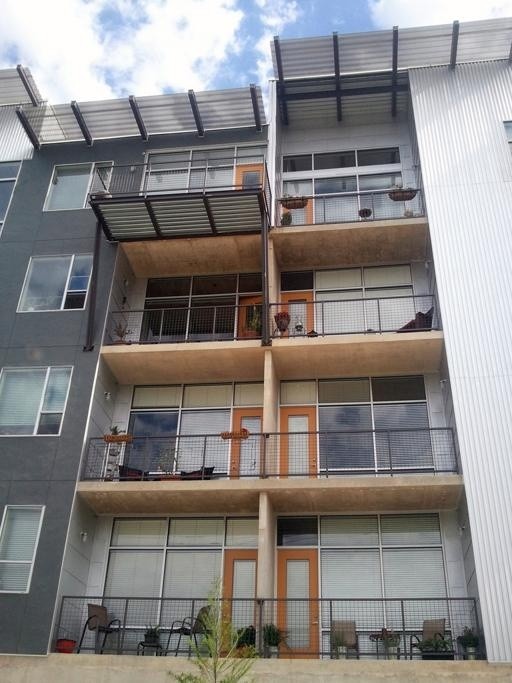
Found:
[111,323,133,345]
[383,635,400,659]
[331,631,350,660]
[416,637,454,653]
[242,306,262,339]
[456,626,479,660]
[141,622,163,641]
[233,622,291,658]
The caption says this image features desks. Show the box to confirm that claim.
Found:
[369,633,403,659]
[135,641,163,656]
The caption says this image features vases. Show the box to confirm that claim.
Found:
[276,320,290,331]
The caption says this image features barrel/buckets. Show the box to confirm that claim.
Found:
[57,638,76,653]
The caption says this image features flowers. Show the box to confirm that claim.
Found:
[276,311,290,321]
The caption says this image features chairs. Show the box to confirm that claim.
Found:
[76,603,121,654]
[117,465,215,480]
[165,606,213,658]
[408,618,446,660]
[330,619,359,659]
